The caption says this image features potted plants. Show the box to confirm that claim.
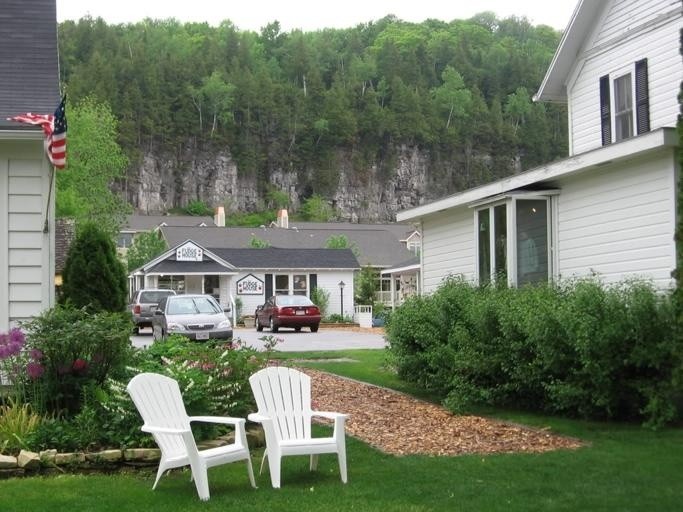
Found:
[331,314,342,323]
[345,316,349,324]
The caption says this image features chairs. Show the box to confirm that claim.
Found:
[126,372,258,500]
[247,366,349,490]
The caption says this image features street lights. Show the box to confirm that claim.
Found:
[338,280,346,320]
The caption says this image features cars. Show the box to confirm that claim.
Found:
[254,294,322,332]
[128,289,233,345]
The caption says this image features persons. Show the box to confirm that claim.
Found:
[518,229,540,278]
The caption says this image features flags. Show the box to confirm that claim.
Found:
[6,93,67,169]
